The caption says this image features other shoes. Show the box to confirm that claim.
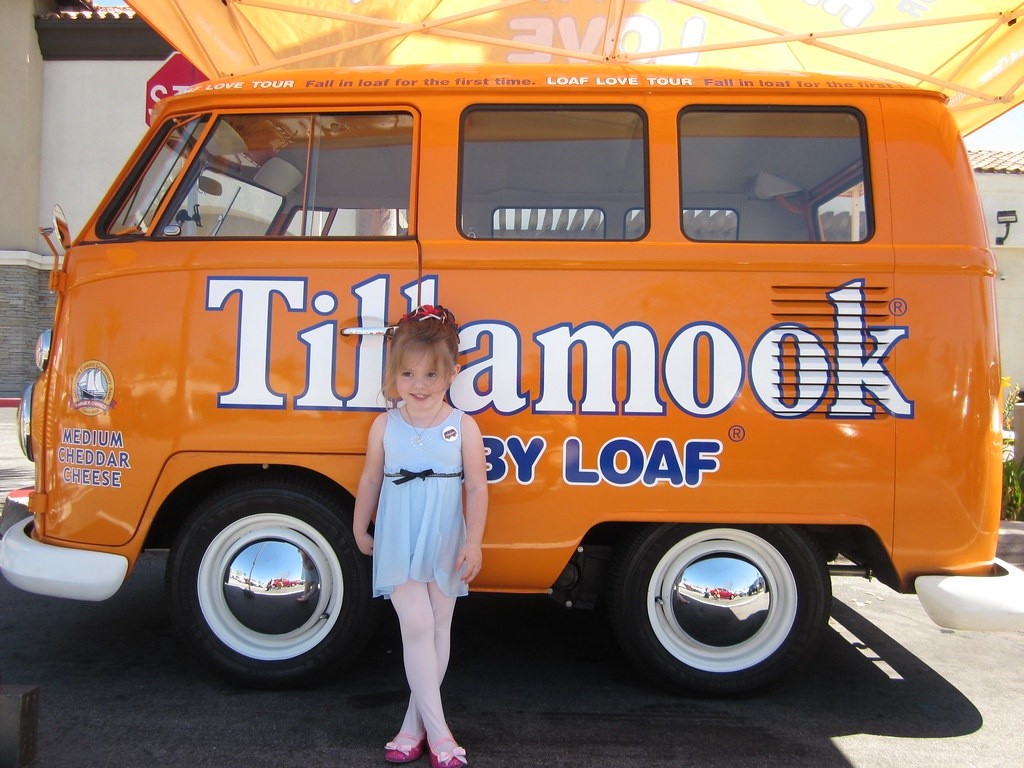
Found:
[296,596,308,603]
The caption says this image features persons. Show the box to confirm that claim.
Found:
[353,307,487,768]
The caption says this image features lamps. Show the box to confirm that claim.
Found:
[995,210,1017,244]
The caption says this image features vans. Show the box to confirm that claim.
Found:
[0,60,1005,701]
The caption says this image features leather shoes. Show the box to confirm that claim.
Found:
[385,730,467,768]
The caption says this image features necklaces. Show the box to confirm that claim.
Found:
[404,403,444,447]
